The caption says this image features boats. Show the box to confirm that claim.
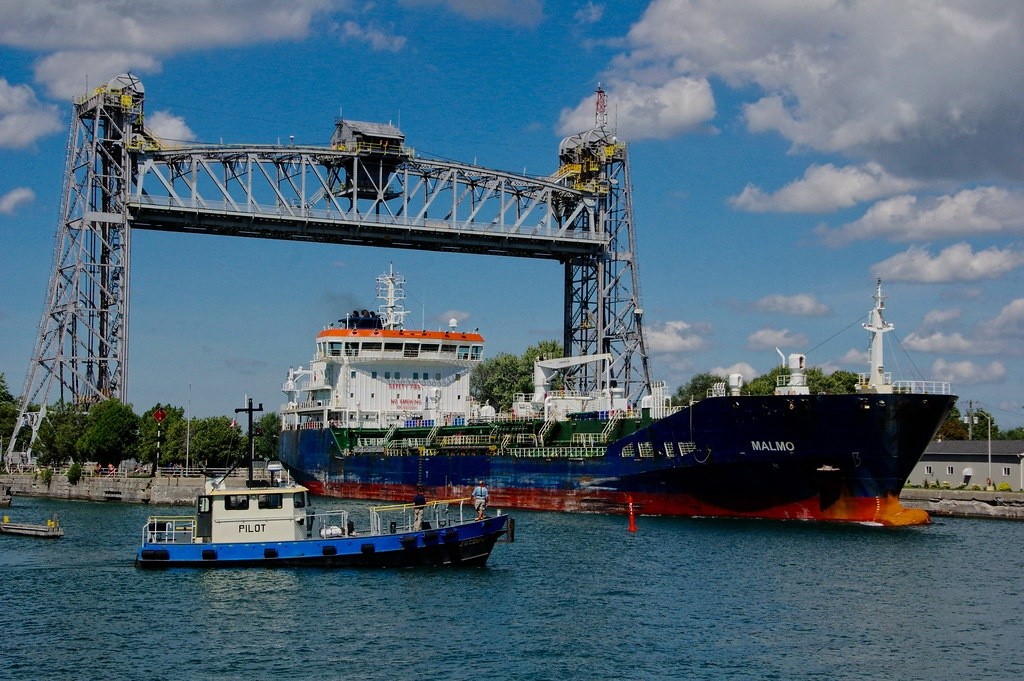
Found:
[0,494,12,508]
[0,513,64,539]
[134,396,507,571]
[277,262,960,528]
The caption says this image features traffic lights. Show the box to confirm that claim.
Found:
[260,429,263,436]
[255,428,259,436]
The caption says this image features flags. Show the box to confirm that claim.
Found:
[230,419,238,428]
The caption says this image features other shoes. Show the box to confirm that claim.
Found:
[475,517,483,521]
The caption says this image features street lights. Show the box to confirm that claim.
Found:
[973,411,992,485]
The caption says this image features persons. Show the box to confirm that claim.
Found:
[412,488,426,532]
[472,481,489,521]
